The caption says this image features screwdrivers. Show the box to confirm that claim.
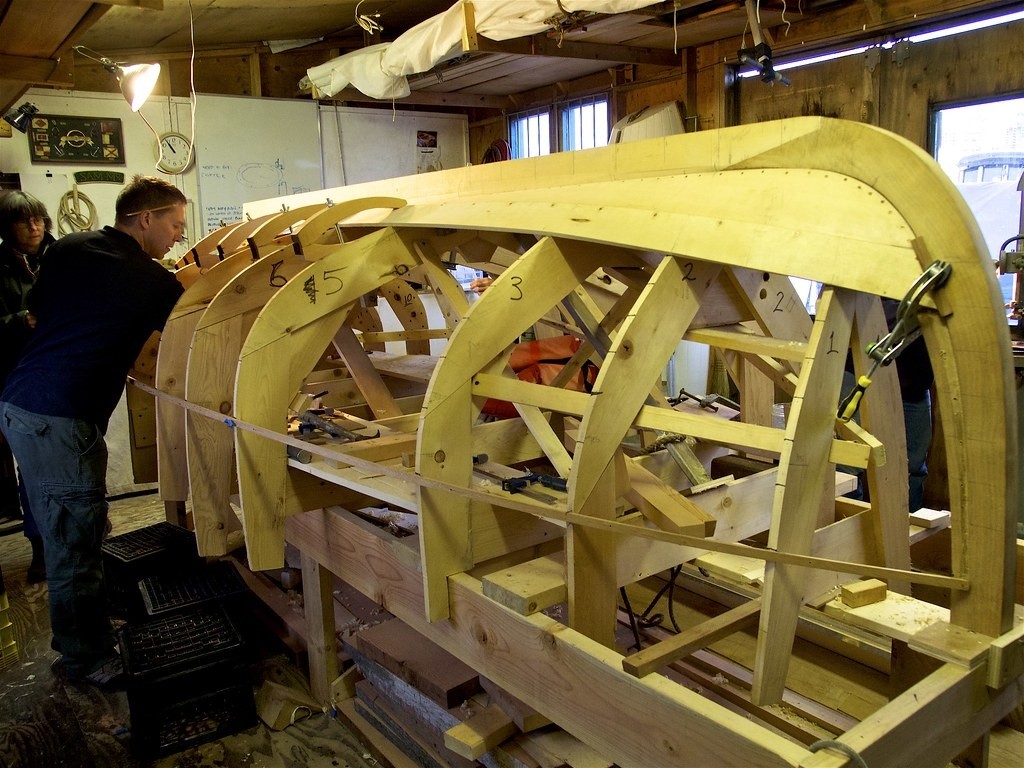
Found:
[836,318,904,423]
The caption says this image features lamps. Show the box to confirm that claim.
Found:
[4,102,39,134]
[73,45,161,112]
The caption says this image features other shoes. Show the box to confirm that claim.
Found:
[51,647,127,692]
[26,537,47,584]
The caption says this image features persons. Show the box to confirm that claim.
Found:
[0,177,187,690]
[0,189,56,341]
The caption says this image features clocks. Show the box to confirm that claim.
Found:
[153,132,195,173]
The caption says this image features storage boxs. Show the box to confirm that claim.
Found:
[100,522,260,762]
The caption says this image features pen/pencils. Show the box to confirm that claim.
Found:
[126,204,176,217]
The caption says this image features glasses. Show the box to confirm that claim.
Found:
[11,216,50,229]
